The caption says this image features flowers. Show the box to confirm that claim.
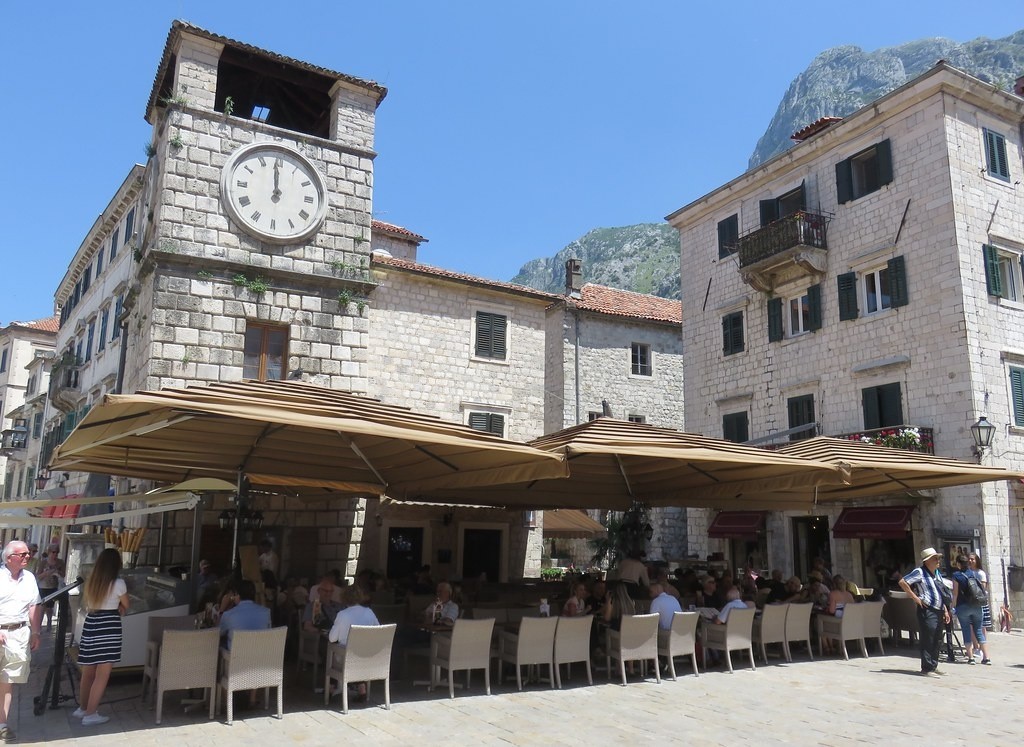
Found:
[811,215,820,228]
[848,427,933,449]
[794,212,805,221]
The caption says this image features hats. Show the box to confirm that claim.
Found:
[807,571,823,580]
[921,547,942,561]
[200,560,211,568]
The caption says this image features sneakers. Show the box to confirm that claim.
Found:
[72,707,109,725]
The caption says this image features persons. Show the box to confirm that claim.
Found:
[951,552,991,665]
[0,537,65,633]
[899,548,950,678]
[563,548,861,680]
[950,545,970,567]
[190,542,467,714]
[0,541,42,741]
[72,548,131,726]
[866,549,915,592]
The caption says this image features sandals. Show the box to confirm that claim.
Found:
[0,727,16,741]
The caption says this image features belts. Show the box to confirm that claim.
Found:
[0,622,26,630]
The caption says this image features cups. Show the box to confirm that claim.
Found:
[122,552,138,569]
[182,572,189,580]
[105,542,121,551]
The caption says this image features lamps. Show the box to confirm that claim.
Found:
[970,416,996,458]
[217,496,263,528]
[34,474,49,489]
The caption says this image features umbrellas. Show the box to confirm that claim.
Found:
[44,381,1024,510]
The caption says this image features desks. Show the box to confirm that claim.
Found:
[408,623,464,691]
[495,621,550,685]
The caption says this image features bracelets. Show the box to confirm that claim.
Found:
[952,608,956,611]
[31,631,42,636]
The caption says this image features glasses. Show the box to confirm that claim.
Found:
[11,552,30,557]
[52,551,59,553]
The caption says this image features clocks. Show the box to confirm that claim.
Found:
[220,141,328,246]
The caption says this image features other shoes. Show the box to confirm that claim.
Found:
[330,695,344,706]
[968,659,975,664]
[981,659,991,664]
[920,671,948,678]
[353,694,366,706]
[965,649,981,657]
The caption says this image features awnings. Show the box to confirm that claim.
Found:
[832,506,913,539]
[543,509,608,539]
[708,510,768,540]
[0,487,116,530]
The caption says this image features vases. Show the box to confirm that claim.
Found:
[813,229,821,245]
[797,220,804,244]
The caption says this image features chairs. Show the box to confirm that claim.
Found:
[297,579,885,714]
[140,614,288,725]
